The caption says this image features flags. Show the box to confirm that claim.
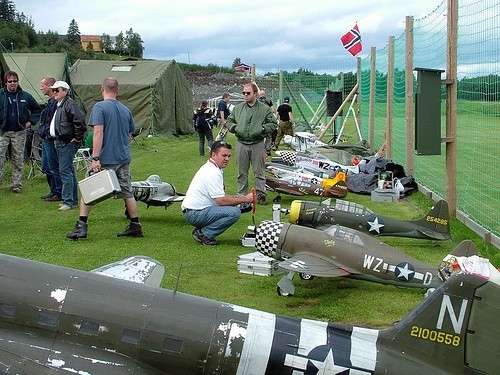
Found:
[340,24,362,57]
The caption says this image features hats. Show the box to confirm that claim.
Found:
[48,81,70,89]
[285,96,290,100]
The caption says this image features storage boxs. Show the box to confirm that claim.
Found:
[371,189,400,204]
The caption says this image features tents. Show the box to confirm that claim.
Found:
[68,59,195,139]
[0,41,72,160]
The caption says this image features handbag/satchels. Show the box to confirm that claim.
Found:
[217,109,230,119]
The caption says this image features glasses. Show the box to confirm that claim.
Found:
[7,81,18,83]
[213,141,230,150]
[52,89,64,92]
[243,92,255,95]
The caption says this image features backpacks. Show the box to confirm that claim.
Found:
[194,108,208,132]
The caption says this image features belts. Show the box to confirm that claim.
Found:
[182,209,190,213]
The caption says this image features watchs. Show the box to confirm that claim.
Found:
[92,156,100,161]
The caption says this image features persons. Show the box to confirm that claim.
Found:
[66,77,144,239]
[258,89,275,156]
[225,83,278,206]
[272,96,296,151]
[216,93,231,142]
[0,71,43,193]
[194,99,215,157]
[47,81,88,211]
[37,77,64,202]
[181,140,254,247]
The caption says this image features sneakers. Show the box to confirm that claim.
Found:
[117,221,144,237]
[207,146,211,151]
[59,203,78,208]
[272,146,277,151]
[46,195,62,201]
[41,193,55,198]
[266,150,271,156]
[258,197,266,204]
[192,230,218,246]
[11,183,21,192]
[66,220,88,238]
[200,154,205,158]
[240,204,253,213]
[57,203,73,211]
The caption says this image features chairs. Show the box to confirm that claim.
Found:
[28,133,47,180]
[72,148,92,171]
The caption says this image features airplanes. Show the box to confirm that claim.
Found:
[0,253,500,375]
[124,175,186,219]
[265,123,404,203]
[247,220,478,297]
[280,197,451,241]
